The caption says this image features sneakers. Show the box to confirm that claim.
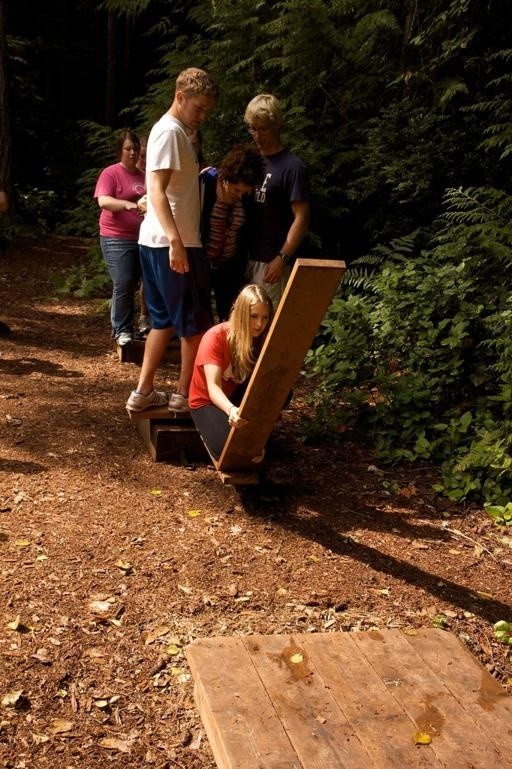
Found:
[167,392,191,414]
[117,333,134,346]
[134,327,149,339]
[125,388,169,413]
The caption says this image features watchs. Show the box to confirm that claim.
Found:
[276,253,289,263]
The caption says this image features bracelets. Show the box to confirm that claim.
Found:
[228,405,237,417]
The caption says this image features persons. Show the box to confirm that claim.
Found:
[93,129,148,347]
[244,92,309,409]
[136,134,153,335]
[125,67,219,415]
[184,128,210,172]
[189,284,276,470]
[198,144,262,326]
